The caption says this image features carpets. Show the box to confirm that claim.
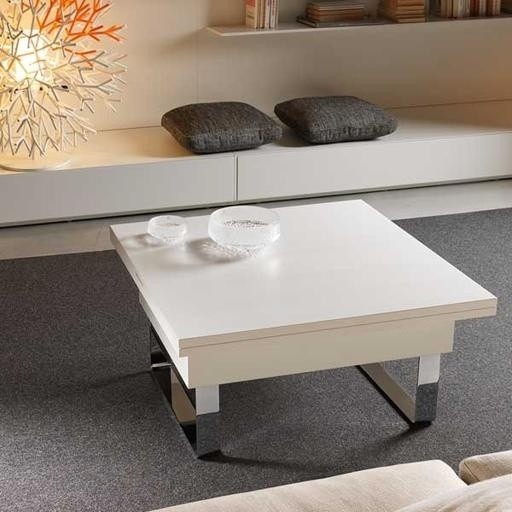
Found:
[0,207,512,512]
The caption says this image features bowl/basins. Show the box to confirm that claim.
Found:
[147,215,187,240]
[208,206,284,248]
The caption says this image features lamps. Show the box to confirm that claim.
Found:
[0,0,126,173]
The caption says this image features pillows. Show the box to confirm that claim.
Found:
[159,100,283,156]
[274,95,400,145]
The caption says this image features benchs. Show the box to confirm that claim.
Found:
[0,98,511,226]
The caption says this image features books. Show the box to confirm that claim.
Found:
[243,1,511,30]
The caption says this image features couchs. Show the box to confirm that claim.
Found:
[143,447,512,512]
